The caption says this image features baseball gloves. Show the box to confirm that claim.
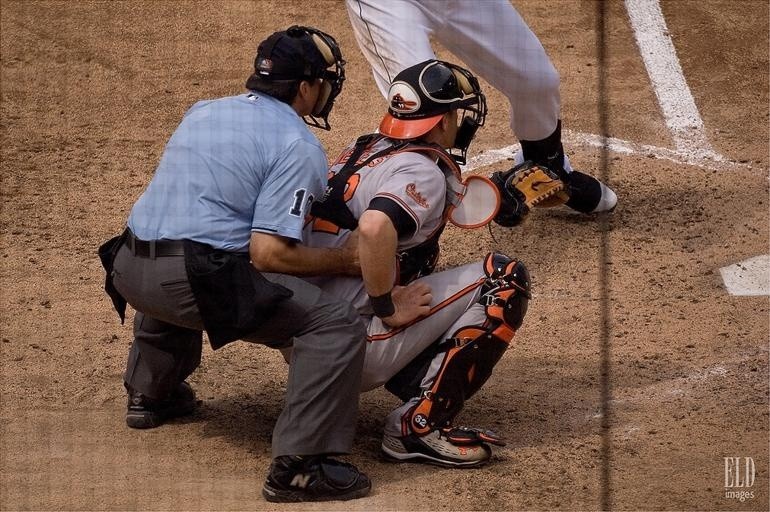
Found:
[489,159,570,226]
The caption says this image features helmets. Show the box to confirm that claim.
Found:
[378,60,484,166]
[244,24,346,131]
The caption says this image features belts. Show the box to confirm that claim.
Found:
[119,227,185,260]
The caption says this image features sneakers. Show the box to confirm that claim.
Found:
[125,381,198,430]
[395,242,440,286]
[379,425,491,469]
[512,143,619,213]
[262,454,372,502]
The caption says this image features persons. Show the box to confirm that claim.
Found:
[97,25,375,503]
[295,57,569,470]
[345,0,619,216]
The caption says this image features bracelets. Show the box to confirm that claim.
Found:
[368,292,396,318]
